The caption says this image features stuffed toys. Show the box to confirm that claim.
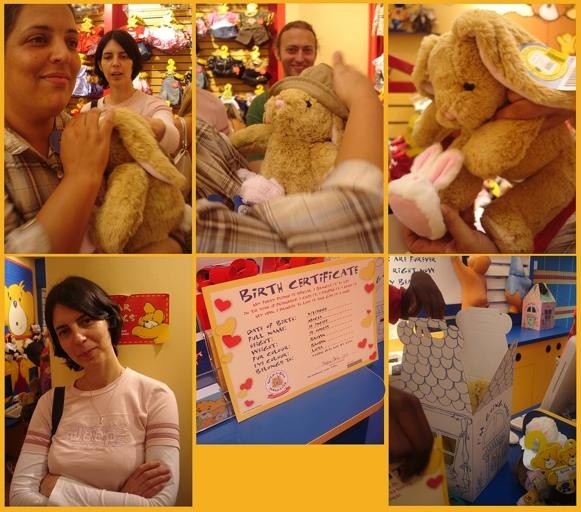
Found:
[451,255,557,331]
[89,107,187,253]
[227,60,350,197]
[388,5,575,252]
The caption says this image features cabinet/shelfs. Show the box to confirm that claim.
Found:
[506,333,567,417]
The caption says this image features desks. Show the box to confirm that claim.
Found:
[195,342,385,444]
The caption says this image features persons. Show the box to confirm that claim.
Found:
[80,28,181,153]
[403,87,575,253]
[4,3,190,253]
[9,274,181,508]
[196,50,384,252]
[389,271,447,485]
[244,20,319,174]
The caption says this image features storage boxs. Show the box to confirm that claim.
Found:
[388,307,520,503]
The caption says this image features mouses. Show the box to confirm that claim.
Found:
[509,431,519,446]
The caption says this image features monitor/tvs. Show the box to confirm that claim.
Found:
[542,334,576,415]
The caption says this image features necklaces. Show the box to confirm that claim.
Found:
[84,368,123,425]
